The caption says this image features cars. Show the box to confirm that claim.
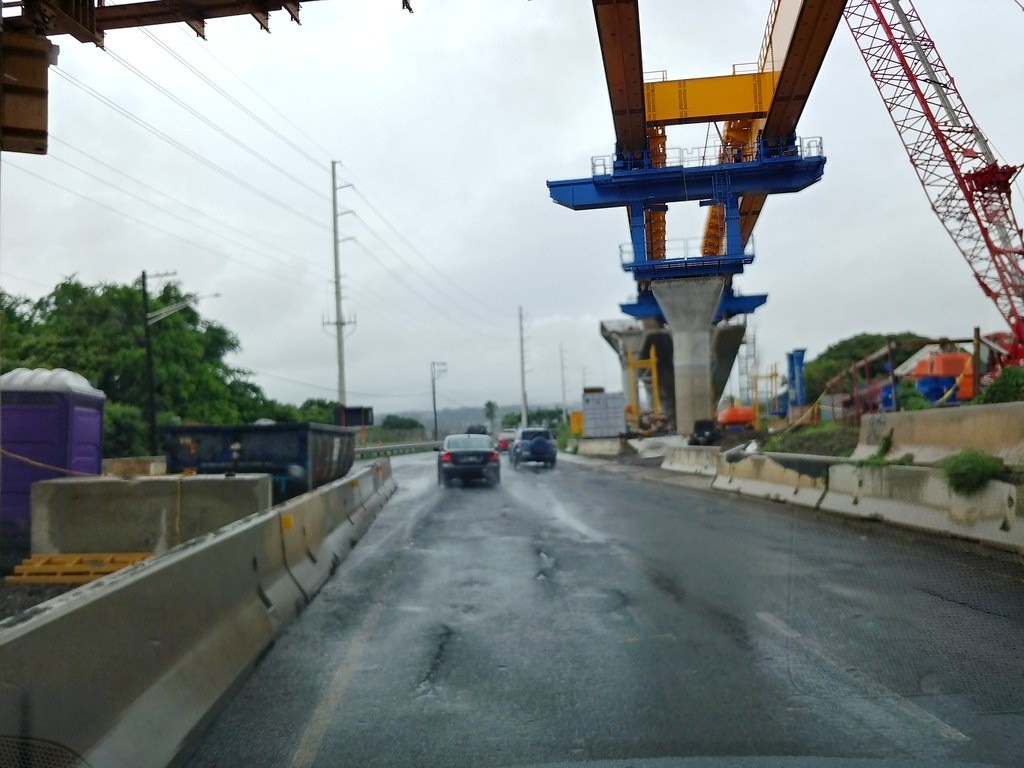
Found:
[497,432,515,452]
[432,431,500,489]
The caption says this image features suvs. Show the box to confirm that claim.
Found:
[508,427,557,470]
